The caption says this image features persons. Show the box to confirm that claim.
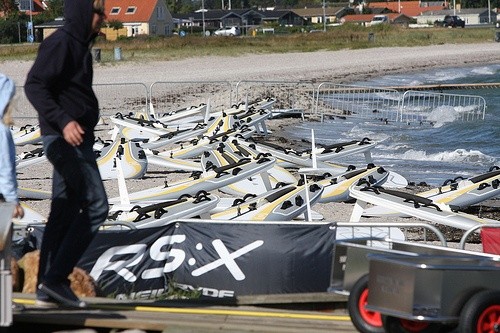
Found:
[24,0,110,309]
[0,73,26,290]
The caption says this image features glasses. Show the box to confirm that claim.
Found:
[93,8,105,15]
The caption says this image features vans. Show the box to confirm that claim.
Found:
[370,15,387,27]
[215,26,240,37]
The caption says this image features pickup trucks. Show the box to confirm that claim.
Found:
[434,15,465,28]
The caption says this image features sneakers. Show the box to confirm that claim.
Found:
[38,279,86,308]
